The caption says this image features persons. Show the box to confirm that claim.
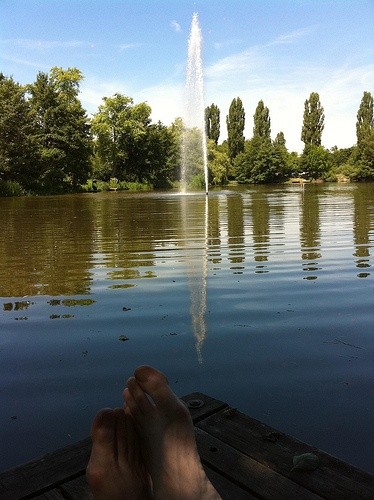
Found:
[85,365,224,499]
